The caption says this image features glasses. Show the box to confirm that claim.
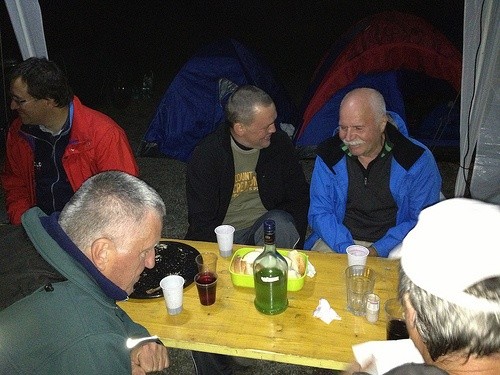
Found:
[8,93,36,107]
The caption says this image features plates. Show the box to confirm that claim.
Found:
[228,248,308,291]
[128,241,203,299]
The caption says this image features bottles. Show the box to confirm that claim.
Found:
[254,220,289,316]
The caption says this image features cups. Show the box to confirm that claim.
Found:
[346,244,370,275]
[383,298,410,340]
[160,275,185,316]
[196,253,218,273]
[194,272,217,306]
[365,294,380,323]
[214,225,236,257]
[345,265,376,315]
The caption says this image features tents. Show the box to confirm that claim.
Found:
[292,7,459,147]
[139,33,301,161]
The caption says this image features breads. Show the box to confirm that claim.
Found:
[232,250,307,278]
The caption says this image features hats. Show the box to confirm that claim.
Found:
[399,197,500,312]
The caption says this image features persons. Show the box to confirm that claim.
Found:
[303,88,443,257]
[184,86,309,251]
[344,198,500,375]
[0,57,138,224]
[0,170,169,375]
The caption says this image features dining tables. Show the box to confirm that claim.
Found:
[116,238,399,372]
